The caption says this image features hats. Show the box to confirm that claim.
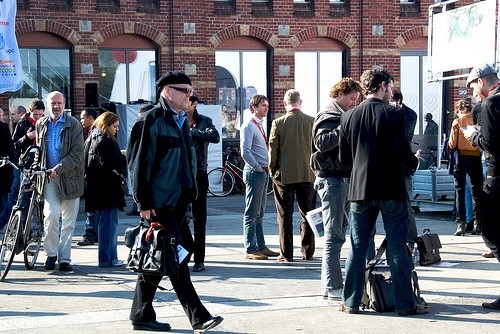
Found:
[466,63,497,87]
[155,71,191,95]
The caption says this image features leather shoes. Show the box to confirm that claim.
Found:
[192,316,223,333]
[132,319,171,331]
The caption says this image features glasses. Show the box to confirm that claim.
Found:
[169,86,193,94]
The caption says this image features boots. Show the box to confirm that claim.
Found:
[454,222,465,236]
[471,221,481,235]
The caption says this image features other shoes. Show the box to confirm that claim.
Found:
[59,263,74,273]
[77,239,92,245]
[277,255,293,262]
[482,301,497,309]
[482,251,494,257]
[260,247,280,256]
[126,211,140,216]
[44,256,57,269]
[115,260,124,266]
[340,304,358,314]
[246,251,268,260]
[193,263,204,272]
[399,304,430,316]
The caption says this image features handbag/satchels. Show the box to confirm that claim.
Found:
[123,216,175,276]
[417,228,442,265]
[369,274,395,311]
[112,170,128,191]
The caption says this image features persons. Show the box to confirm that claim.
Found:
[183,95,220,271]
[268,63,500,314]
[0,92,157,334]
[221,104,241,142]
[240,95,280,261]
[424,113,438,135]
[126,70,225,334]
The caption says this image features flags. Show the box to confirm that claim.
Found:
[0,0,24,95]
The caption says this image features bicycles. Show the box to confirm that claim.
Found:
[207,147,273,197]
[0,144,51,282]
